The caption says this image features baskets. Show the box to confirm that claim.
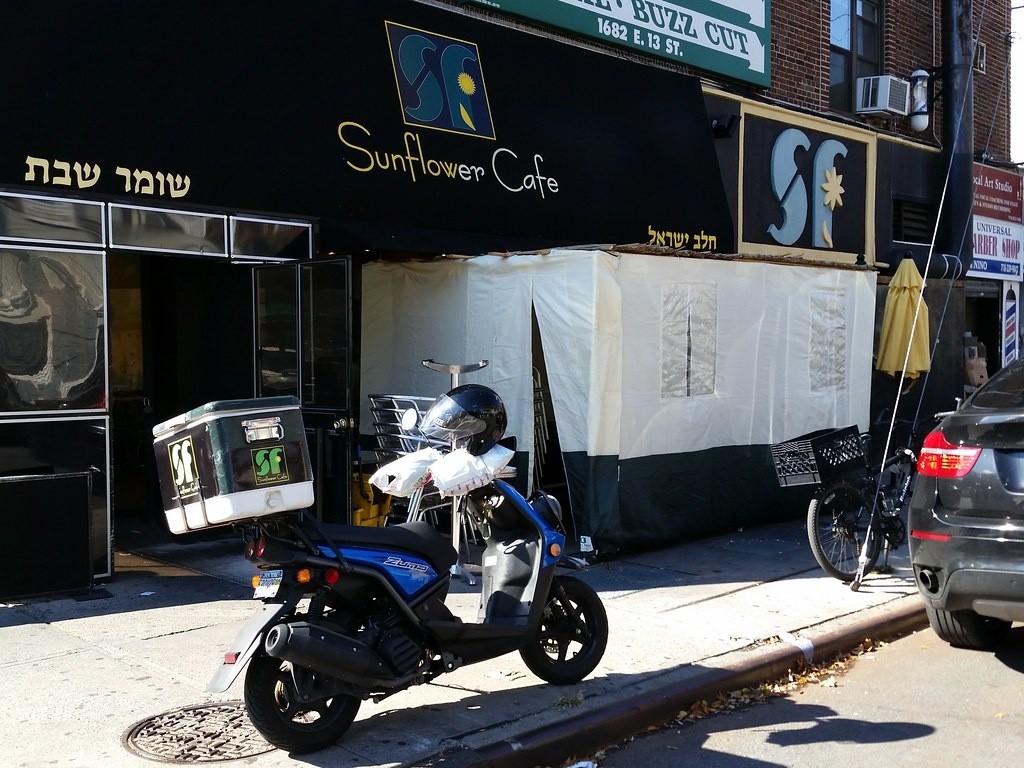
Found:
[770,425,866,487]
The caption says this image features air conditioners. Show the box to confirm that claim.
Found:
[856,75,910,119]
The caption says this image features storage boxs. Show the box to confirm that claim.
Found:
[152,395,315,535]
[769,424,867,487]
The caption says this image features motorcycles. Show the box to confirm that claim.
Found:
[151,408,609,755]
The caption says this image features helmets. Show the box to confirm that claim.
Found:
[435,384,507,457]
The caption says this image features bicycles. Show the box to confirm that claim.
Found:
[771,410,958,582]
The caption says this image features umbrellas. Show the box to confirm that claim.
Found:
[875,258,931,380]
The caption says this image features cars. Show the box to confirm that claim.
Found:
[908,355,1024,650]
